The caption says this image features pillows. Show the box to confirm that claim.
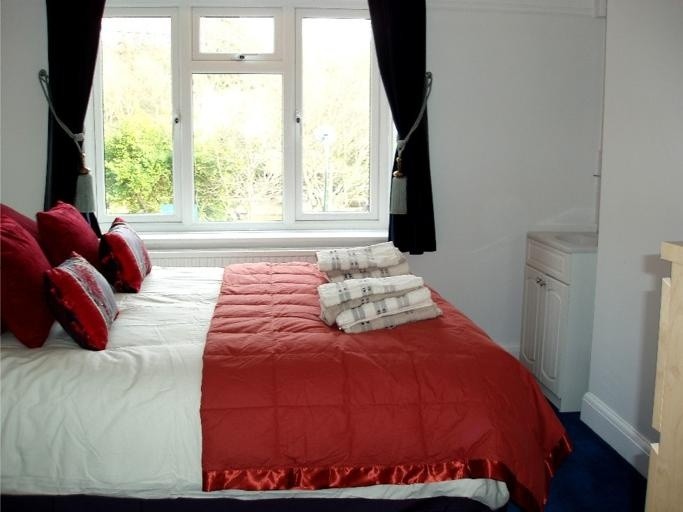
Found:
[94,215,153,296]
[48,251,121,351]
[0,204,63,349]
[0,200,39,245]
[33,200,99,264]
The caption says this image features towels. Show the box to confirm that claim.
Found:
[309,239,444,336]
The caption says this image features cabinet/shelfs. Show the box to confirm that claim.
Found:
[519,232,598,412]
[645,242,683,511]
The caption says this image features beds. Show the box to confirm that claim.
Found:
[0,263,575,510]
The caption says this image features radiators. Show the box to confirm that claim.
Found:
[143,244,324,272]
